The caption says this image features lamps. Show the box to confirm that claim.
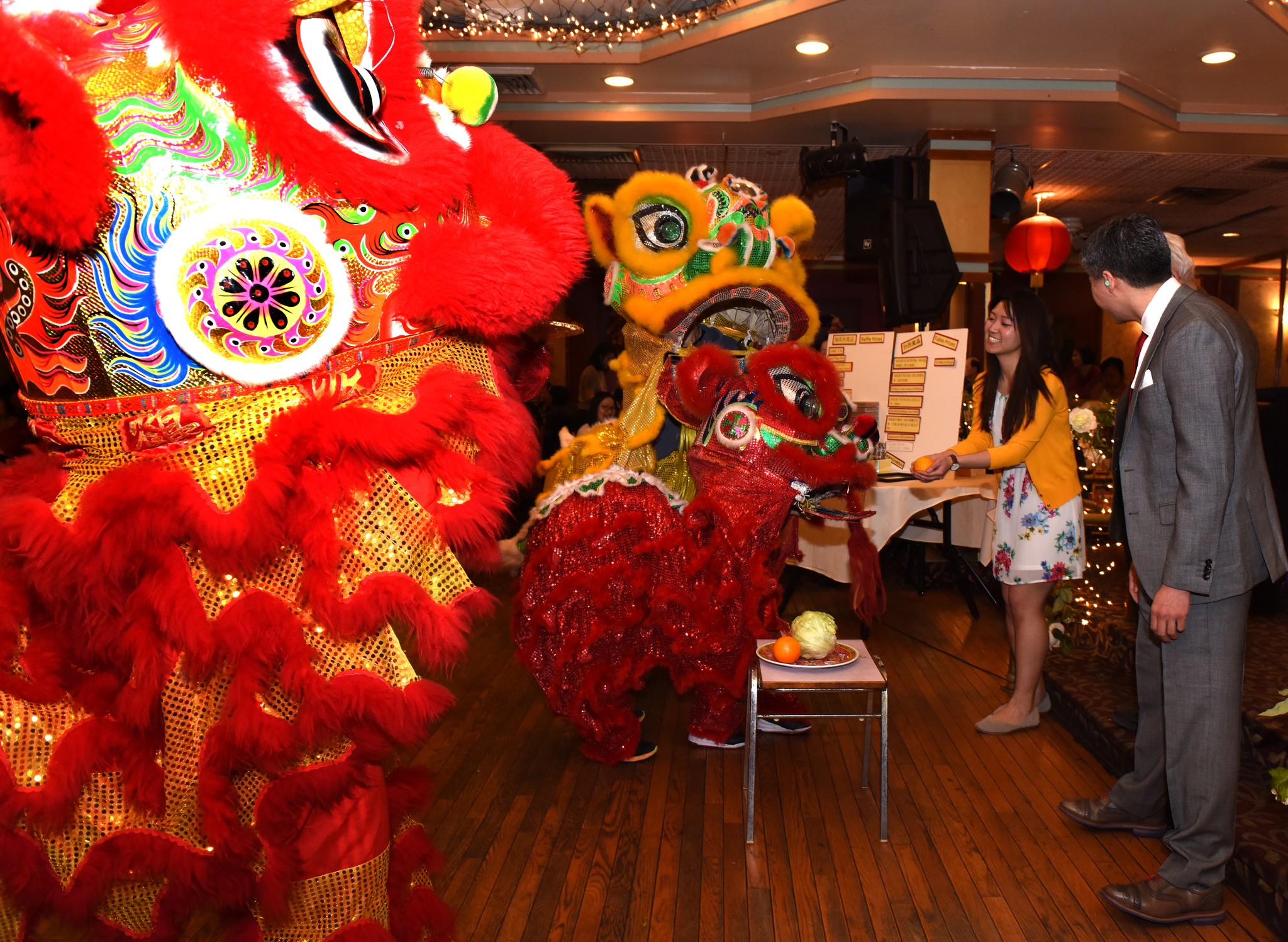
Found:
[990,144,1034,220]
[799,119,867,189]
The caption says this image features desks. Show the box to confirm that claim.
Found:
[786,471,999,620]
[743,638,887,848]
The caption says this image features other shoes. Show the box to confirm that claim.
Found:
[755,718,812,734]
[975,706,1040,735]
[993,692,1051,715]
[631,708,646,722]
[622,738,658,762]
[687,732,746,748]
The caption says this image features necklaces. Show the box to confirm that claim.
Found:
[697,326,700,339]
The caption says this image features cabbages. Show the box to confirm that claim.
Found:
[790,610,838,659]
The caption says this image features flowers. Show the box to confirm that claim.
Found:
[1067,406,1114,460]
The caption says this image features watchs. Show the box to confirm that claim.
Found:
[947,454,960,471]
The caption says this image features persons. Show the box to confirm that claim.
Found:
[1058,213,1288,925]
[910,289,1086,734]
[543,385,577,459]
[614,387,623,417]
[649,317,740,460]
[1090,358,1128,402]
[576,341,618,431]
[576,391,616,435]
[1062,345,1101,399]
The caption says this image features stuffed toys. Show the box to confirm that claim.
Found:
[0,0,588,942]
[500,165,821,566]
[510,339,878,765]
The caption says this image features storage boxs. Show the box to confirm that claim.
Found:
[955,469,986,477]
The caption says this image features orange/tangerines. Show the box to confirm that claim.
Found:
[913,456,934,472]
[773,636,802,663]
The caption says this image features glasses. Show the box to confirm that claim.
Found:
[600,404,617,412]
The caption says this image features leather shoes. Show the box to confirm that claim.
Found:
[1057,793,1168,838]
[1099,874,1227,925]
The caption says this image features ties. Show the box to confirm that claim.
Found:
[1127,331,1148,416]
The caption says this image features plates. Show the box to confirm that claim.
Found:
[756,642,859,669]
